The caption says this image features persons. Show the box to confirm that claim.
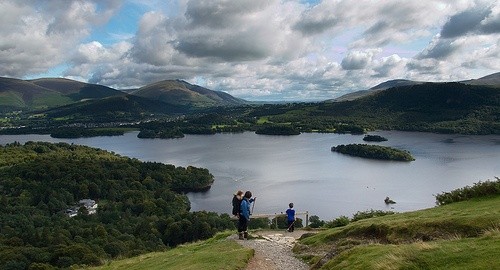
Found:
[286,203,296,232]
[238,191,256,239]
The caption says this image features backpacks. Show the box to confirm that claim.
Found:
[232,194,247,215]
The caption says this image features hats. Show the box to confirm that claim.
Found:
[244,191,252,198]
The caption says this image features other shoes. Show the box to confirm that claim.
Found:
[244,232,249,238]
[288,230,293,232]
[239,233,244,239]
